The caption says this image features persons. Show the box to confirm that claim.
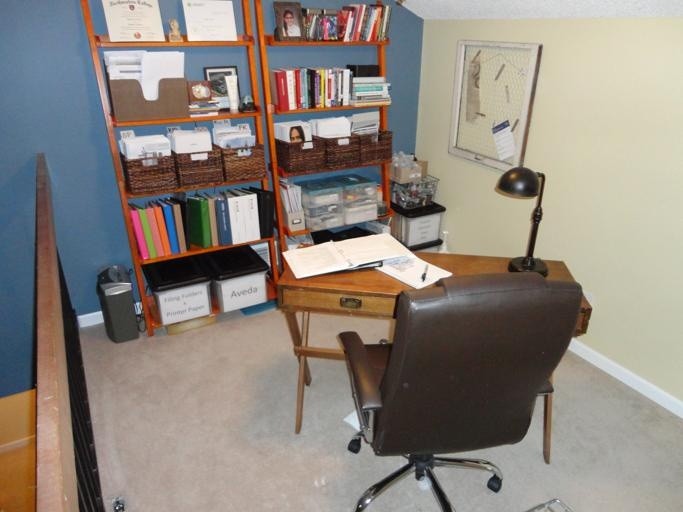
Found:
[289,126,305,143]
[282,10,302,38]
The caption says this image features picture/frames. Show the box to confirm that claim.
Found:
[203,66,242,113]
[274,1,307,41]
[447,38,542,175]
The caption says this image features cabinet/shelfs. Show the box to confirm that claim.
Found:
[254,0,395,273]
[81,0,281,338]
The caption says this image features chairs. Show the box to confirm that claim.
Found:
[336,273,584,512]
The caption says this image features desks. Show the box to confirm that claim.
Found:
[275,243,593,466]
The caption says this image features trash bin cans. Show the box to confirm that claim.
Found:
[97,282,139,342]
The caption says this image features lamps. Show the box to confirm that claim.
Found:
[494,167,549,278]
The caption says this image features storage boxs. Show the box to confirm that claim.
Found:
[141,254,212,327]
[388,150,446,253]
[293,178,345,232]
[202,244,270,313]
[325,173,378,226]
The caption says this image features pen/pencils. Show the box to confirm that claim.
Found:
[422,264,428,283]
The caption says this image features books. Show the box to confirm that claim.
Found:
[269,64,392,109]
[304,2,392,42]
[280,231,411,280]
